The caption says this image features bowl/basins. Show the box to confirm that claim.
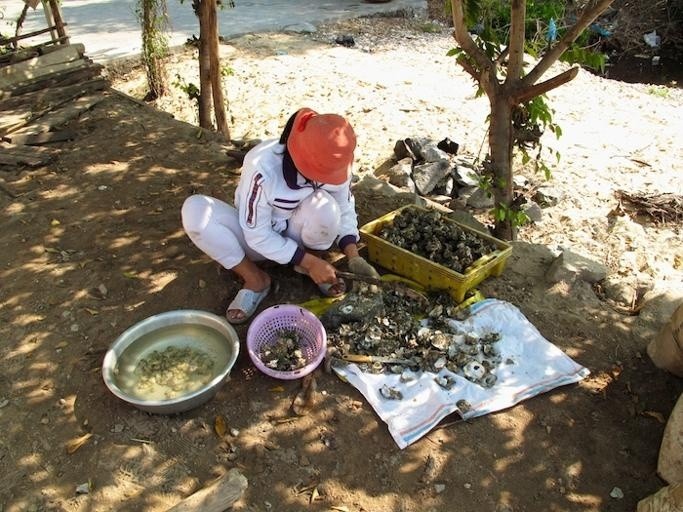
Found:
[101,309,240,414]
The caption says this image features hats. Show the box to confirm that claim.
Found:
[287,108,356,185]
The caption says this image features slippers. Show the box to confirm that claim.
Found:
[315,268,345,296]
[225,280,271,324]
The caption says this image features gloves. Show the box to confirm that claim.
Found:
[349,258,380,292]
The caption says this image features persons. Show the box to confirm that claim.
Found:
[181,108,381,324]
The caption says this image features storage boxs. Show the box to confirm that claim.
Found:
[359,204,512,304]
